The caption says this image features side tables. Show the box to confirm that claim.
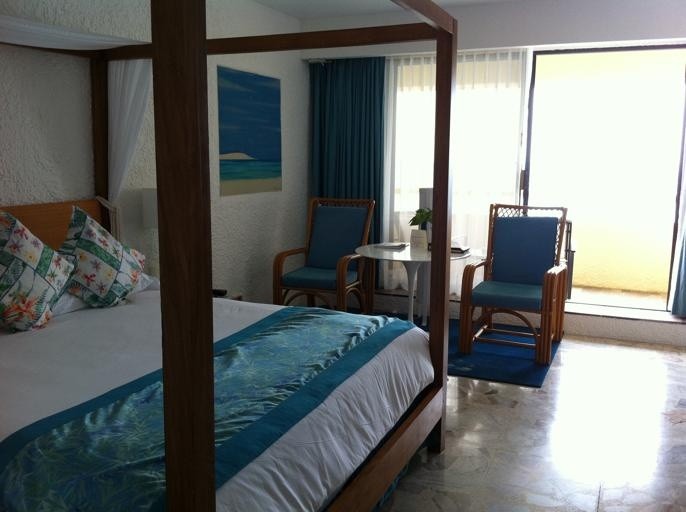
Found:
[351,242,471,326]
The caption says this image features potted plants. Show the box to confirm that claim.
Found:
[406,207,434,248]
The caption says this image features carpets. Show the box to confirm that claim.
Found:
[353,311,565,388]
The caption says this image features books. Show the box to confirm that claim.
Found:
[451,247,469,254]
[373,241,405,250]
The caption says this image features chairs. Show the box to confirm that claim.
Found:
[456,201,569,362]
[269,194,376,315]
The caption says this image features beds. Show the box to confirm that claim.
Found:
[0,202,448,511]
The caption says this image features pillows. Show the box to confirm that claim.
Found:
[0,203,153,333]
